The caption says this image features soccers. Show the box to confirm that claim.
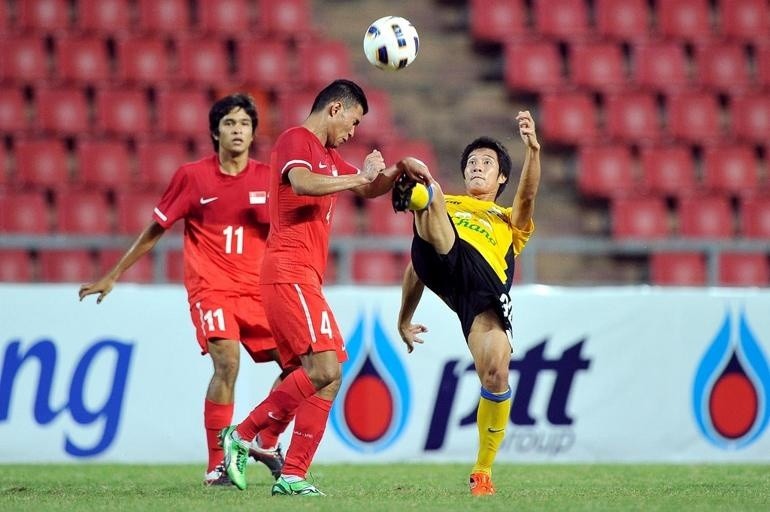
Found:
[363,16,419,73]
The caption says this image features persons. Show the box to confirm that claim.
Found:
[394,109,541,495]
[216,78,432,497]
[78,93,303,486]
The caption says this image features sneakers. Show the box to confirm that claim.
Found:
[272,475,324,497]
[392,171,416,213]
[249,435,285,479]
[469,472,495,495]
[203,469,236,488]
[217,425,250,491]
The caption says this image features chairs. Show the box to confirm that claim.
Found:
[1,3,769,287]
